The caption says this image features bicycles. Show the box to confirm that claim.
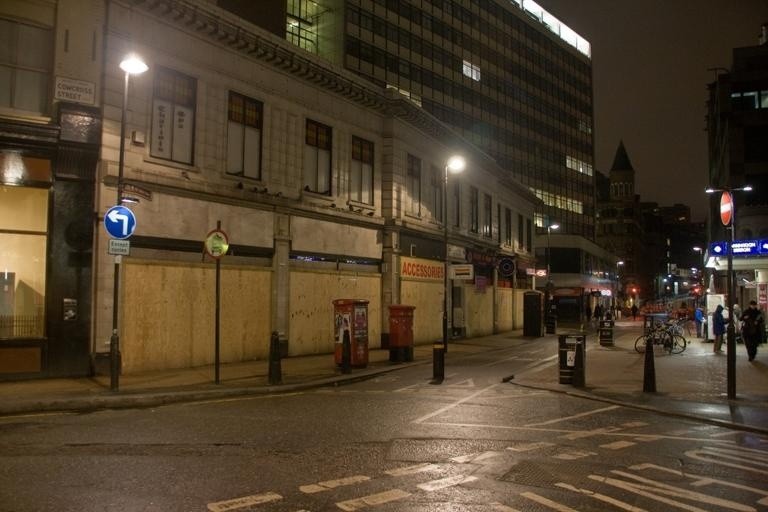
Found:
[633,316,690,355]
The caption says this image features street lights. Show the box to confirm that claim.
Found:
[615,261,624,319]
[105,53,148,391]
[693,247,703,314]
[546,223,560,334]
[703,185,753,403]
[441,154,464,352]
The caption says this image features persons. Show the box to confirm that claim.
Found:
[738,300,763,362]
[593,304,600,322]
[694,305,705,338]
[631,304,637,321]
[584,303,591,322]
[712,304,729,352]
[599,304,605,319]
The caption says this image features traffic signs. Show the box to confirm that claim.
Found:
[119,182,153,201]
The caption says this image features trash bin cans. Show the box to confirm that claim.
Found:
[600,320,614,346]
[558,334,586,385]
[644,316,653,328]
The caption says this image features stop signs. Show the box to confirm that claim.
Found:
[720,191,733,226]
